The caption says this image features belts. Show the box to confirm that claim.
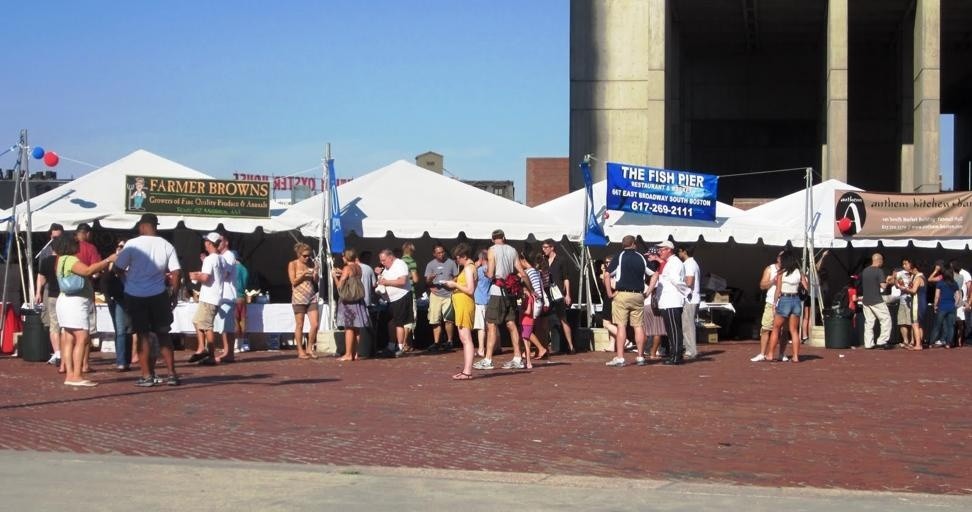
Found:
[618,289,643,293]
[781,293,799,297]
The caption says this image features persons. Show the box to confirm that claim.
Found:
[749,251,789,362]
[764,249,809,363]
[35,214,249,388]
[831,252,972,351]
[445,242,479,381]
[332,230,576,369]
[801,250,829,340]
[594,236,701,366]
[287,242,320,359]
[131,178,147,210]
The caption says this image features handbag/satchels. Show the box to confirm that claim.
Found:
[549,280,565,303]
[542,290,552,312]
[905,296,912,308]
[60,272,85,294]
[651,287,659,317]
[798,283,808,300]
[444,302,455,322]
[494,274,522,295]
[337,278,365,302]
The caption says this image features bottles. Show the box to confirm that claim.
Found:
[265,290,270,303]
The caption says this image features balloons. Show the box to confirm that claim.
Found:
[43,152,59,167]
[839,218,852,232]
[31,147,44,160]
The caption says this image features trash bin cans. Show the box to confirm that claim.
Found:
[551,309,580,353]
[822,308,853,349]
[20,309,49,362]
[359,307,378,359]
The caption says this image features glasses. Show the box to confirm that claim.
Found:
[303,254,310,258]
[116,244,123,249]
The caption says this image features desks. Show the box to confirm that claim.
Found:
[94,301,330,352]
[571,302,737,322]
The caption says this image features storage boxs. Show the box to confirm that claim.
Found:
[590,328,610,352]
[696,325,721,344]
[316,329,345,356]
[707,288,731,303]
[701,273,728,289]
[811,326,826,347]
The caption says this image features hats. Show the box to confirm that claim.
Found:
[203,232,222,246]
[492,230,506,241]
[139,214,160,225]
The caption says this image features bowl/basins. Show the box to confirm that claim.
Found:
[255,296,267,303]
